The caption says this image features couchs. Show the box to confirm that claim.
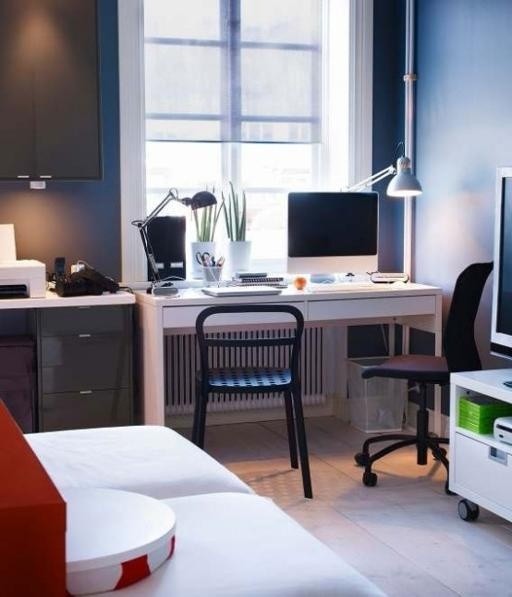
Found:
[1,397,388,597]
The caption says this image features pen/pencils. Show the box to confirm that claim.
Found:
[202,255,225,288]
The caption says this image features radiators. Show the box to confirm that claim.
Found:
[163,321,327,414]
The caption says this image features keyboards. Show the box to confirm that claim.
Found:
[305,283,386,293]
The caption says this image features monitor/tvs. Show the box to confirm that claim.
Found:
[471,164,512,388]
[286,191,378,284]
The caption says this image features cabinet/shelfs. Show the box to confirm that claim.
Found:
[0,1,105,185]
[447,369,512,524]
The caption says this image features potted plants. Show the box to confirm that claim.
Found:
[221,180,252,279]
[187,183,225,278]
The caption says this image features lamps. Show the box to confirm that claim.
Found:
[131,188,217,294]
[337,143,423,285]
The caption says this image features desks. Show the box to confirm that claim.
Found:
[0,281,135,434]
[128,278,444,440]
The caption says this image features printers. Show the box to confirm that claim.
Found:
[0,224,47,299]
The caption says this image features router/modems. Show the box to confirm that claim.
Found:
[371,273,409,283]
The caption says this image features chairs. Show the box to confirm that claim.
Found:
[354,260,493,522]
[191,304,312,499]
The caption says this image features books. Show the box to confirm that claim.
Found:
[224,271,288,289]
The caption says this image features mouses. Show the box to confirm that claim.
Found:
[391,281,407,288]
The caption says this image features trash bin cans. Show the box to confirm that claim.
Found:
[345,357,408,434]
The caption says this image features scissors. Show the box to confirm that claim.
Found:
[196,252,210,266]
